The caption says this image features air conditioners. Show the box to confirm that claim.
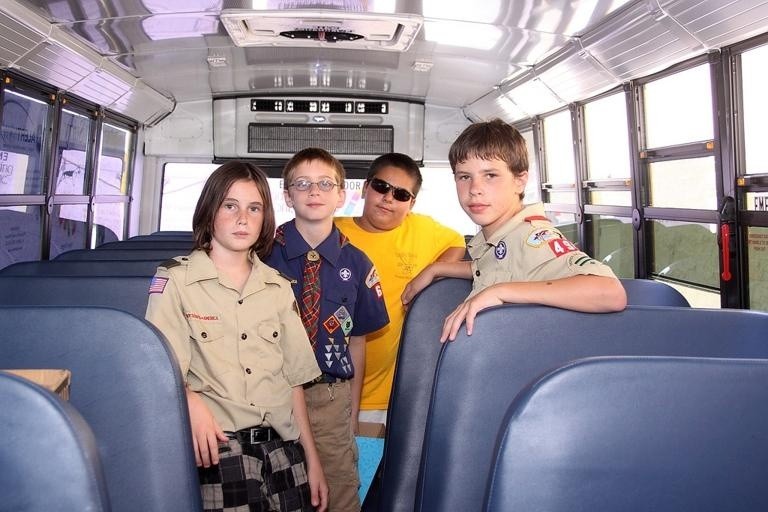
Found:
[211,94,424,163]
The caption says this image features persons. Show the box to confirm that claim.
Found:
[264,147,390,512]
[144,160,329,512]
[333,153,467,508]
[401,120,627,342]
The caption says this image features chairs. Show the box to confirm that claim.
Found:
[128,235,194,241]
[0,276,154,317]
[1,372,115,512]
[151,231,193,235]
[95,240,195,249]
[0,303,202,512]
[380,278,692,512]
[0,260,166,276]
[53,248,191,261]
[415,303,768,511]
[482,354,768,510]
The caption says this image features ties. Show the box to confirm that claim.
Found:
[301,252,321,354]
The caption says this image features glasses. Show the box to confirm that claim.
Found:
[285,178,341,193]
[371,178,415,202]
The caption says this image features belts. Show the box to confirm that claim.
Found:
[233,427,279,443]
[310,374,345,382]
